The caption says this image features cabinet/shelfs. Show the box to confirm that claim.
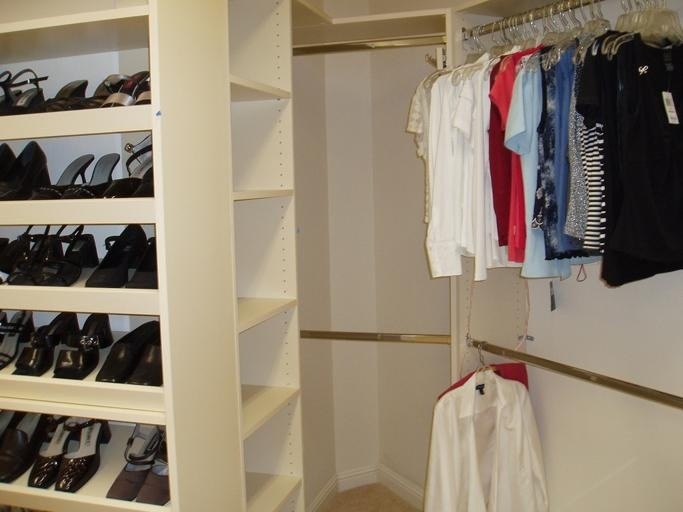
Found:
[1,0,308,512]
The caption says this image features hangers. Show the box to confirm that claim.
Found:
[422,0,682,92]
[471,340,497,375]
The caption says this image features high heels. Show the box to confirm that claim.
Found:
[0,134,155,200]
[1,68,152,115]
[1,411,169,506]
[1,223,158,287]
[0,309,164,387]
